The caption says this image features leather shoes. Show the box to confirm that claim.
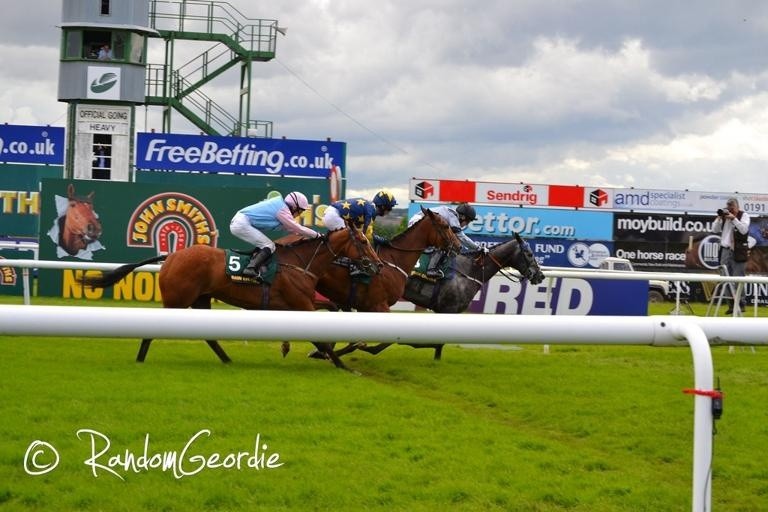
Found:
[725,307,745,314]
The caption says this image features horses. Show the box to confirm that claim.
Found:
[279,204,463,360]
[395,230,546,359]
[58,183,104,255]
[74,218,384,377]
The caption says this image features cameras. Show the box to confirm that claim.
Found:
[717,208,729,216]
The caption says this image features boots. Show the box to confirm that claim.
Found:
[242,247,272,283]
[426,248,448,277]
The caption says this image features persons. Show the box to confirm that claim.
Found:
[230,191,325,282]
[93,141,110,179]
[408,204,490,278]
[322,190,399,276]
[712,198,751,315]
[97,45,112,60]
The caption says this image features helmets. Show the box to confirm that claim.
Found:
[284,191,308,212]
[373,190,399,206]
[455,203,477,223]
